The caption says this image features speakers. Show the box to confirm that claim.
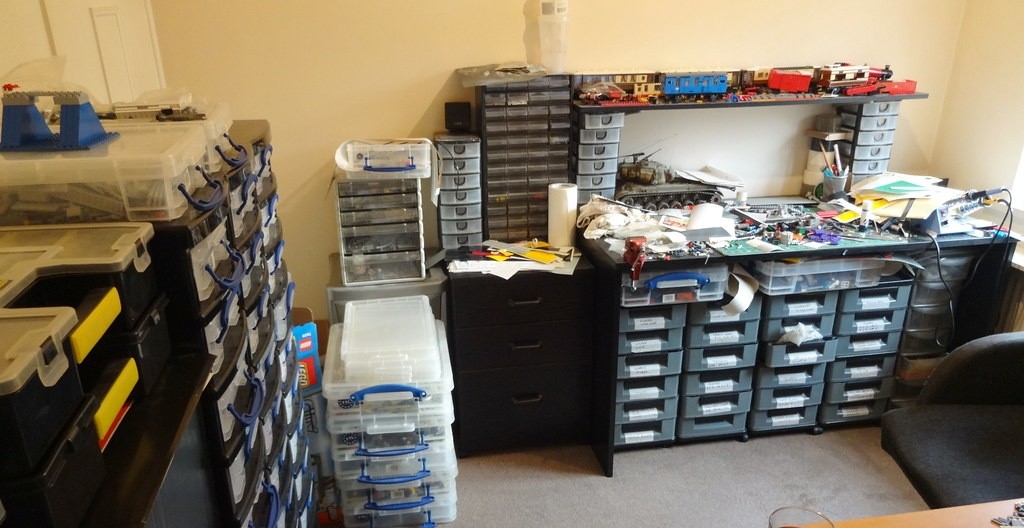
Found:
[444,102,471,135]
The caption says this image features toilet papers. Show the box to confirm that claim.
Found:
[548,182,578,247]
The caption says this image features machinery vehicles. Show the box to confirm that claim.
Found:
[614,148,724,214]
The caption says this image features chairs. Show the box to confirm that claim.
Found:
[880,331,1024,510]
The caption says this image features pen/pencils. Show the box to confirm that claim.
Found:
[820,141,834,175]
[843,165,850,176]
[833,144,842,176]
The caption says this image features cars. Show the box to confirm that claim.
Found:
[581,82,622,102]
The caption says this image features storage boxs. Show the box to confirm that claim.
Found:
[0,294,175,528]
[0,220,158,480]
[306,394,343,528]
[621,259,728,308]
[347,143,431,179]
[58,108,248,175]
[743,257,887,296]
[0,121,228,225]
[290,307,324,397]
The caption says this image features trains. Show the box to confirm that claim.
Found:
[659,72,727,103]
[767,66,869,93]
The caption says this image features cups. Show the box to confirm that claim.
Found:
[822,169,849,201]
[769,506,836,528]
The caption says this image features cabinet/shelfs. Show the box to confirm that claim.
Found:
[0,68,1024,528]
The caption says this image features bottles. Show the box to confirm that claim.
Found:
[736,177,747,207]
[538,0,574,73]
